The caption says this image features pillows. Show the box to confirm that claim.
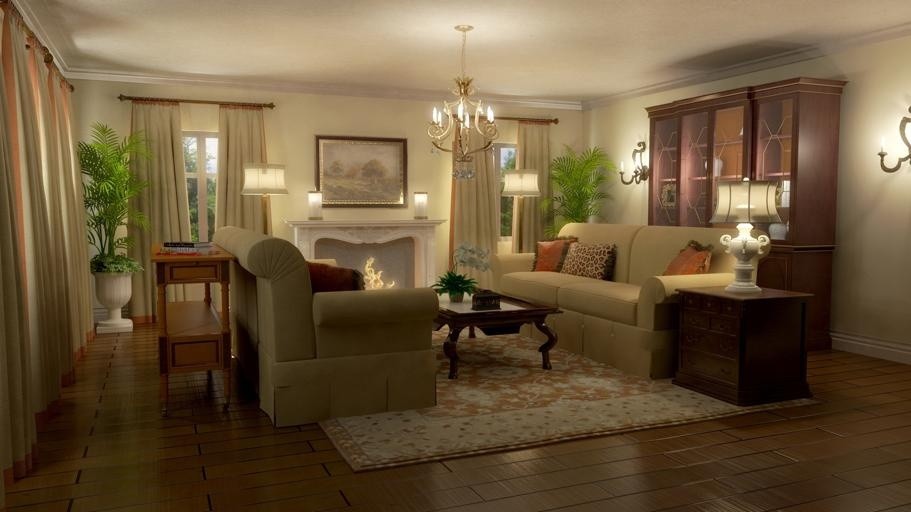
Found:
[662,238,713,275]
[531,236,577,271]
[558,243,616,279]
[308,260,364,290]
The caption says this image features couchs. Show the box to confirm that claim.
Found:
[488,222,771,378]
[213,225,440,424]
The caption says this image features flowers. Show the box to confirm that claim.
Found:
[432,241,491,298]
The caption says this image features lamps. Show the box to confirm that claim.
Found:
[618,139,649,185]
[414,193,429,219]
[502,171,541,254]
[242,161,287,234]
[707,177,783,292]
[426,25,500,165]
[878,106,911,177]
[308,191,323,224]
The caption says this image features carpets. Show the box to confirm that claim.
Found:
[315,322,819,471]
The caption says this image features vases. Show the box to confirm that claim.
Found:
[449,290,465,303]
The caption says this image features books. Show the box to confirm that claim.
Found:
[160,240,214,255]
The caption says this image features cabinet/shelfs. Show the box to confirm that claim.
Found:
[671,284,817,408]
[758,246,836,353]
[645,75,847,248]
[152,243,235,418]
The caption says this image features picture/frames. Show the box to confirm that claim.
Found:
[314,134,408,210]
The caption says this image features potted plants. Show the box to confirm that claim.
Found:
[73,122,160,334]
